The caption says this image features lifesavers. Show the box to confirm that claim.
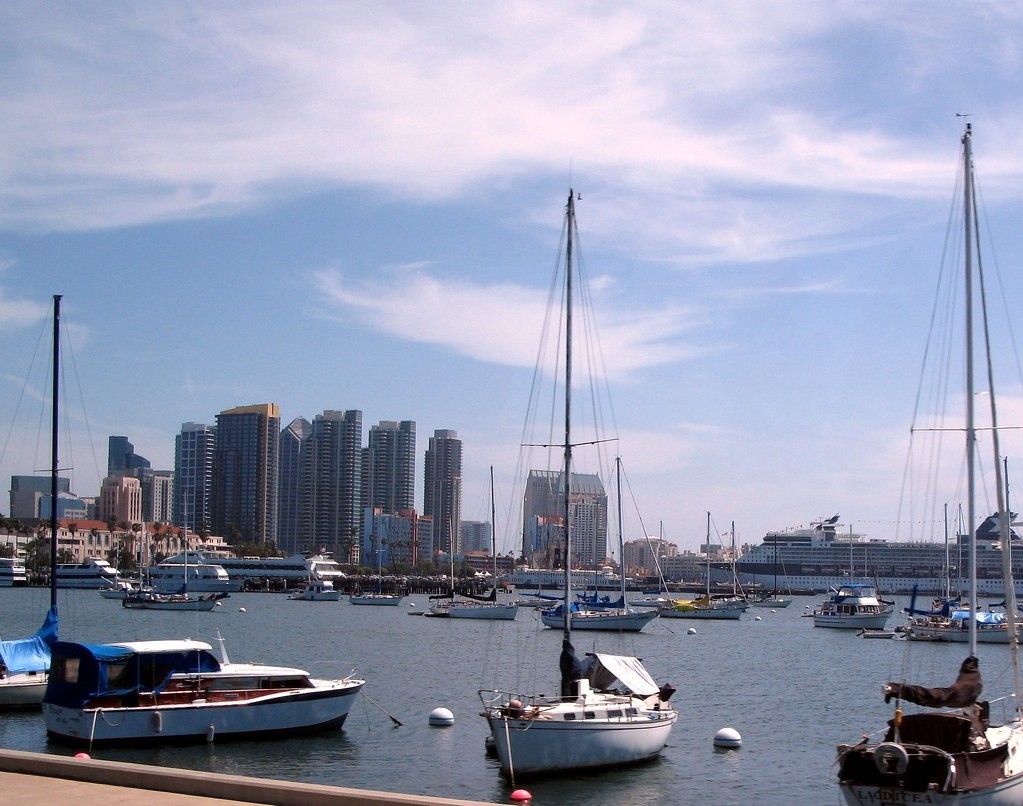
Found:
[873,741,910,775]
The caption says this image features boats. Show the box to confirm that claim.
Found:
[0,557,28,588]
[55,556,141,590]
[41,634,370,748]
[286,568,343,602]
[147,548,245,592]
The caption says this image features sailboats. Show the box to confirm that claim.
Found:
[469,186,678,786]
[425,455,1023,643]
[826,108,1022,806]
[347,507,406,606]
[0,293,126,707]
[98,491,232,611]
